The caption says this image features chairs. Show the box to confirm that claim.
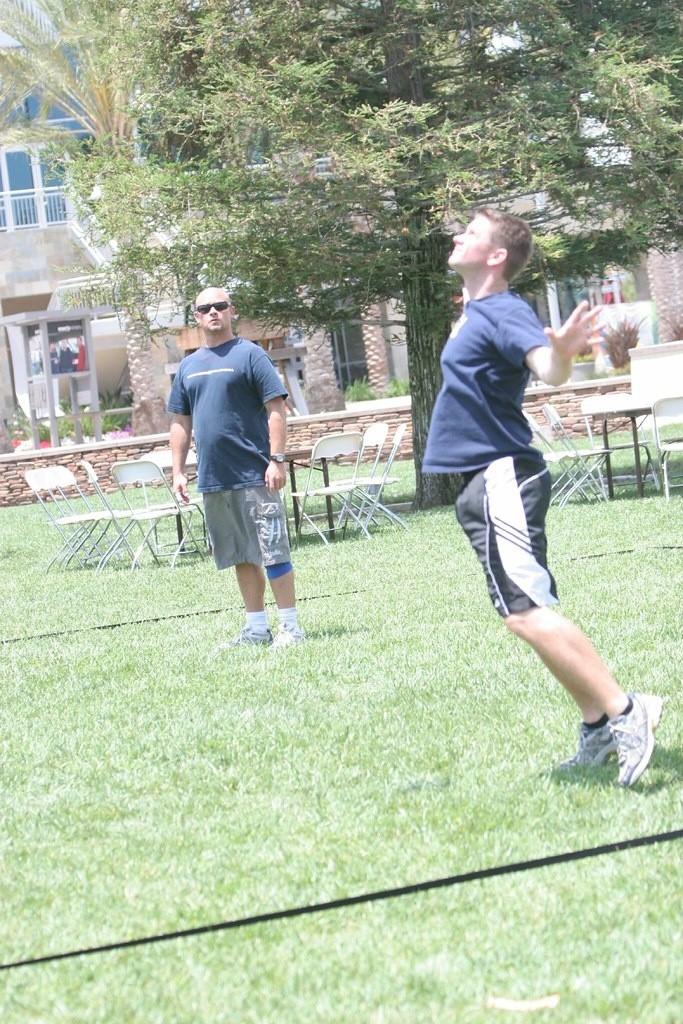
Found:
[521,396,683,508]
[24,421,407,572]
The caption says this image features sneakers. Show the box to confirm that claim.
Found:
[268,621,304,653]
[605,691,665,786]
[560,719,616,772]
[217,627,273,653]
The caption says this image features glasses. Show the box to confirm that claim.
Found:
[197,301,232,315]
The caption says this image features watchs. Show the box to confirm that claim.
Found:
[270,454,286,463]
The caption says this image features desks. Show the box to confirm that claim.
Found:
[175,449,335,553]
[592,406,653,499]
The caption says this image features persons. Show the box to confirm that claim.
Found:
[420,209,662,788]
[168,287,306,649]
[34,337,86,374]
